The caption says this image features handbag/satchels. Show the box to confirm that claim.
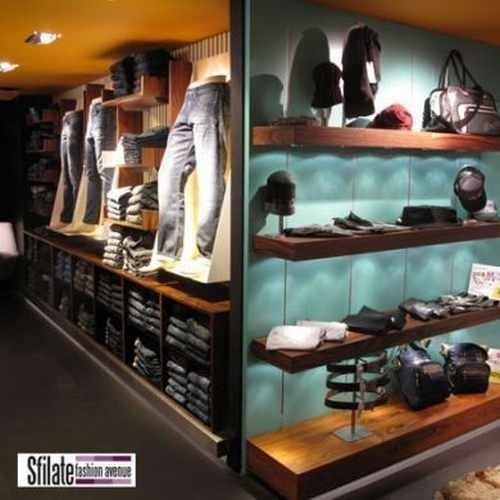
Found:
[425,50,500,136]
[389,338,450,412]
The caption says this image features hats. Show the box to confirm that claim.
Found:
[368,104,414,131]
[308,63,343,109]
[264,169,296,216]
[453,166,488,212]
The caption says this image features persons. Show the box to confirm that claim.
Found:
[64,97,118,234]
[47,107,84,230]
[136,75,232,279]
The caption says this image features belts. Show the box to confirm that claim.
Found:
[327,373,389,392]
[327,350,387,374]
[324,390,391,410]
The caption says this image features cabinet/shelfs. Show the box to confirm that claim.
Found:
[21,226,230,458]
[27,60,191,233]
[252,124,500,500]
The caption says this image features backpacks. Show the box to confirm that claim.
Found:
[445,341,491,396]
